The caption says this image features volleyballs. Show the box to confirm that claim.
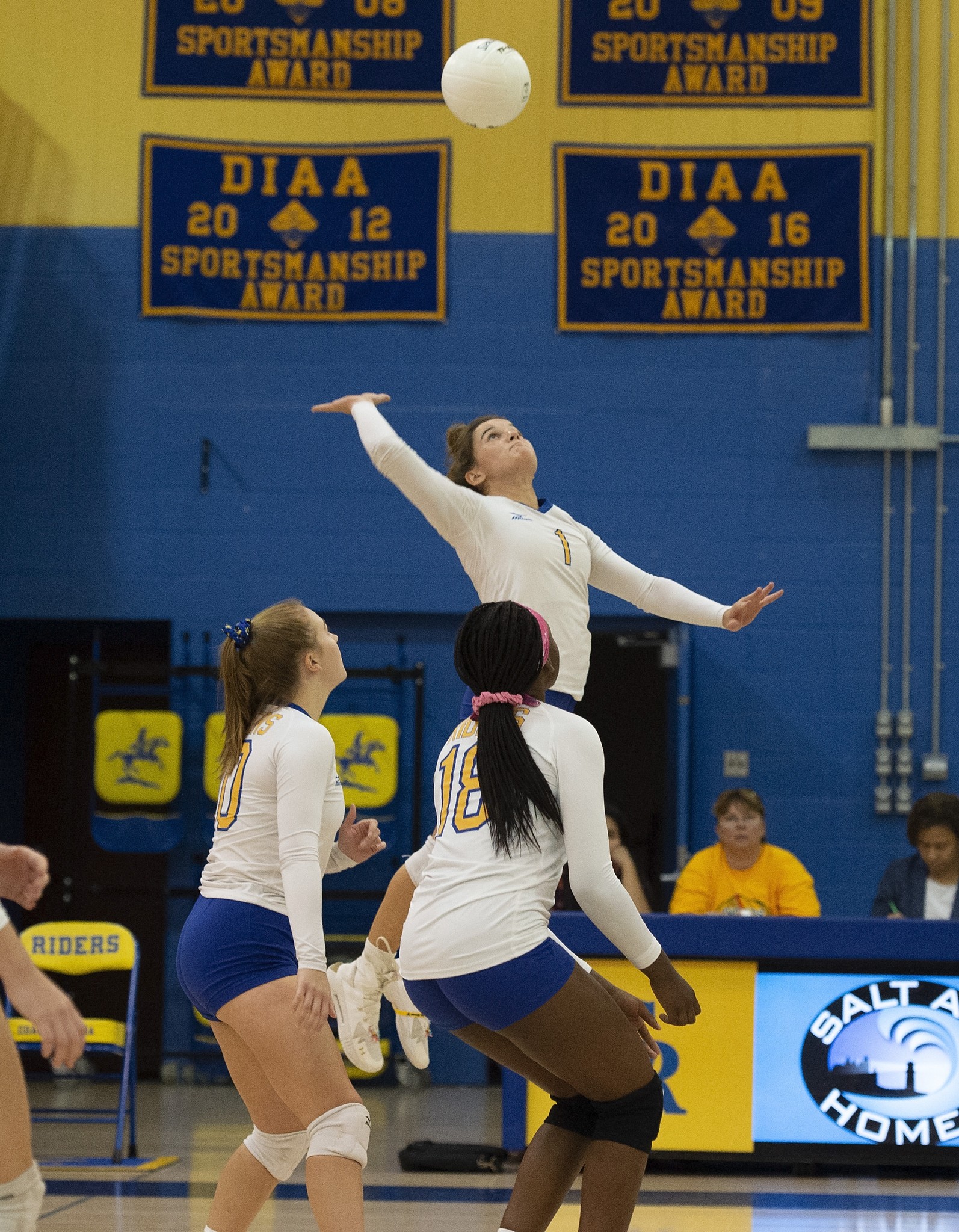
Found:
[440,38,530,129]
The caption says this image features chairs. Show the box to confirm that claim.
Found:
[6,920,139,1164]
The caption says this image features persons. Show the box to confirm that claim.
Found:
[873,792,959,919]
[397,601,702,1232]
[176,597,388,1232]
[311,393,789,1074]
[670,787,822,917]
[552,811,653,915]
[0,838,89,1232]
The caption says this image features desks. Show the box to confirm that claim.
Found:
[501,912,959,1176]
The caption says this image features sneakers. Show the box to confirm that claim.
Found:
[329,950,385,1073]
[382,958,433,1070]
